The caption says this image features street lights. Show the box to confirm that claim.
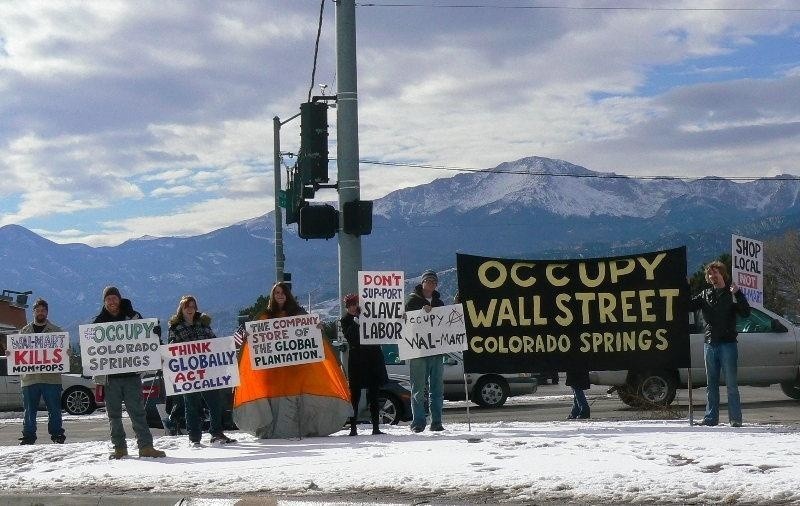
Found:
[305,287,320,315]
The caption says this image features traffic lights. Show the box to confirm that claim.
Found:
[301,204,336,240]
[298,182,317,208]
[344,200,371,235]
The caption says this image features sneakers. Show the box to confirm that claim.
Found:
[411,423,427,432]
[138,444,166,458]
[52,436,66,445]
[696,415,720,427]
[109,446,129,460]
[428,423,446,432]
[728,416,742,428]
[210,436,238,445]
[18,437,36,447]
[189,439,203,449]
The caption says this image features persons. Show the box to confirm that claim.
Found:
[241,281,322,336]
[400,268,445,432]
[167,295,238,448]
[565,369,590,419]
[5,297,71,445]
[686,260,750,428]
[91,286,166,460]
[340,293,388,436]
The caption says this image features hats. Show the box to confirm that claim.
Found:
[420,269,438,284]
[101,285,120,303]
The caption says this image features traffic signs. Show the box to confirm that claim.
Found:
[278,190,287,209]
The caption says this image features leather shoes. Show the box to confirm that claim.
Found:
[372,430,387,436]
[348,429,359,437]
[565,412,592,420]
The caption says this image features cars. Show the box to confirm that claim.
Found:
[143,362,427,432]
[382,344,541,410]
[534,370,560,385]
[588,298,800,409]
[1,354,105,416]
